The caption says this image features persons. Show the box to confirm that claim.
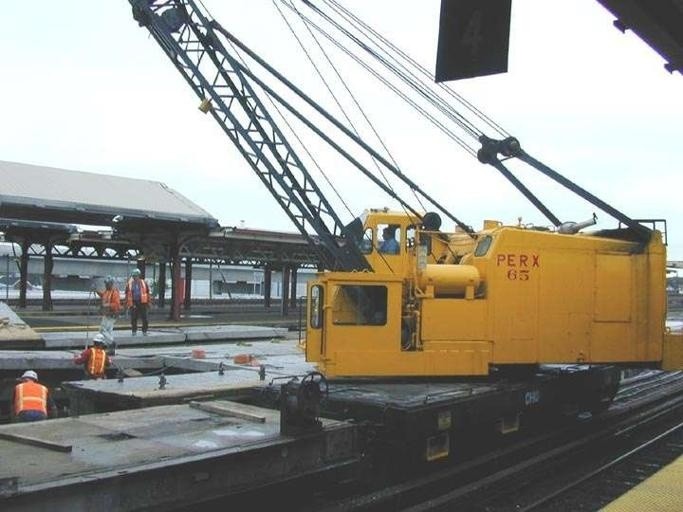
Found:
[125,268,153,336]
[11,369,54,420]
[378,227,400,255]
[73,333,112,379]
[95,276,120,334]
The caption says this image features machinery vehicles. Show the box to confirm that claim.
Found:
[125,0,668,460]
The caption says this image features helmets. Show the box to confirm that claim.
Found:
[22,370,38,382]
[132,268,141,276]
[92,334,105,344]
